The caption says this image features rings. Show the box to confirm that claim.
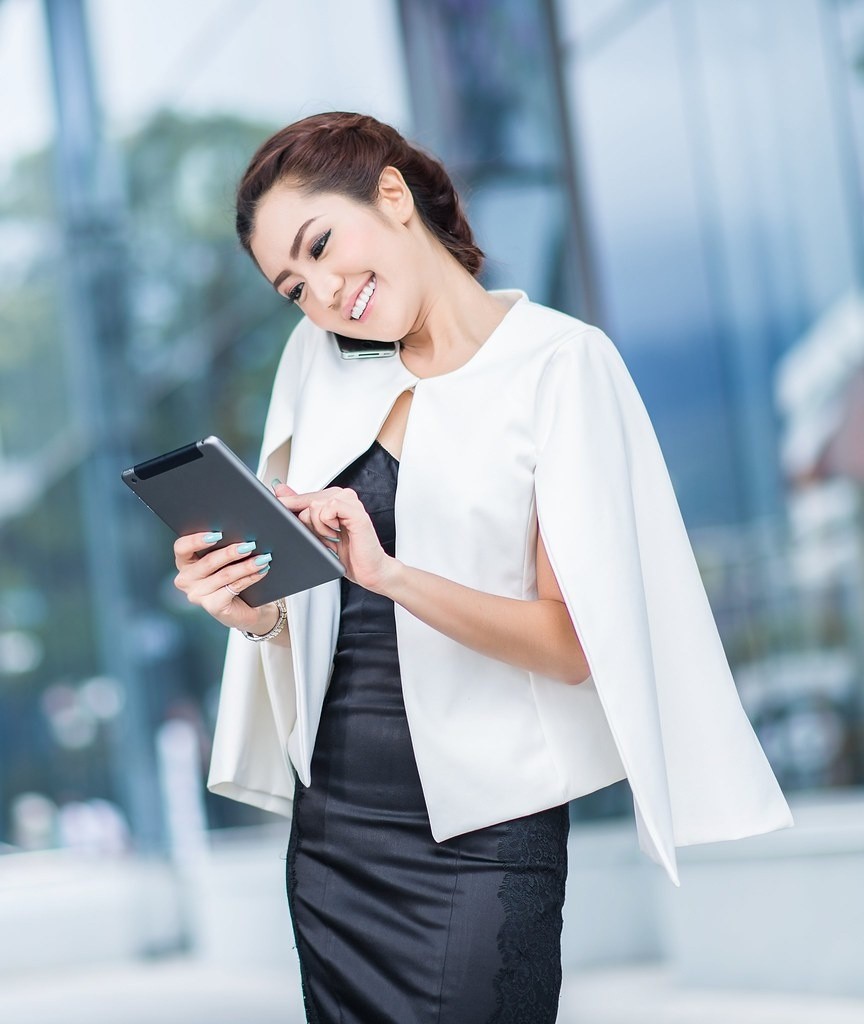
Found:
[226,585,239,596]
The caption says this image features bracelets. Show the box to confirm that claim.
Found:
[241,600,287,643]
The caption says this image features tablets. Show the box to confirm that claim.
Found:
[121,436,346,608]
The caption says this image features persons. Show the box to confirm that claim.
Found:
[172,111,793,1024]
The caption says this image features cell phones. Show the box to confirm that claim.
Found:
[331,330,397,361]
